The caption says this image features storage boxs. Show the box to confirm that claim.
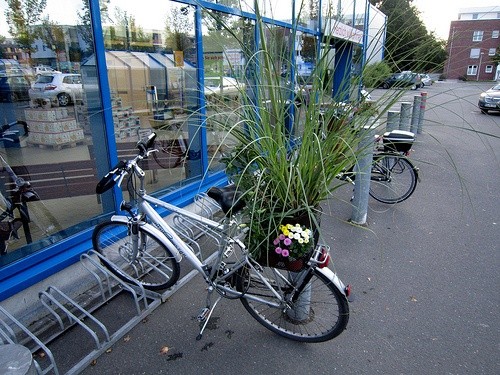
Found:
[23,90,140,145]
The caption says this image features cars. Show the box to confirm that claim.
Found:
[374,70,433,90]
[478,84,500,114]
[0,66,86,107]
[294,73,323,98]
[203,77,247,104]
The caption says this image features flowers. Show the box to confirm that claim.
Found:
[273,223,313,260]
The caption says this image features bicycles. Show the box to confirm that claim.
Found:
[0,120,66,256]
[149,104,240,174]
[287,100,422,204]
[92,132,351,343]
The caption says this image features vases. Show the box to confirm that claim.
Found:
[285,259,304,272]
